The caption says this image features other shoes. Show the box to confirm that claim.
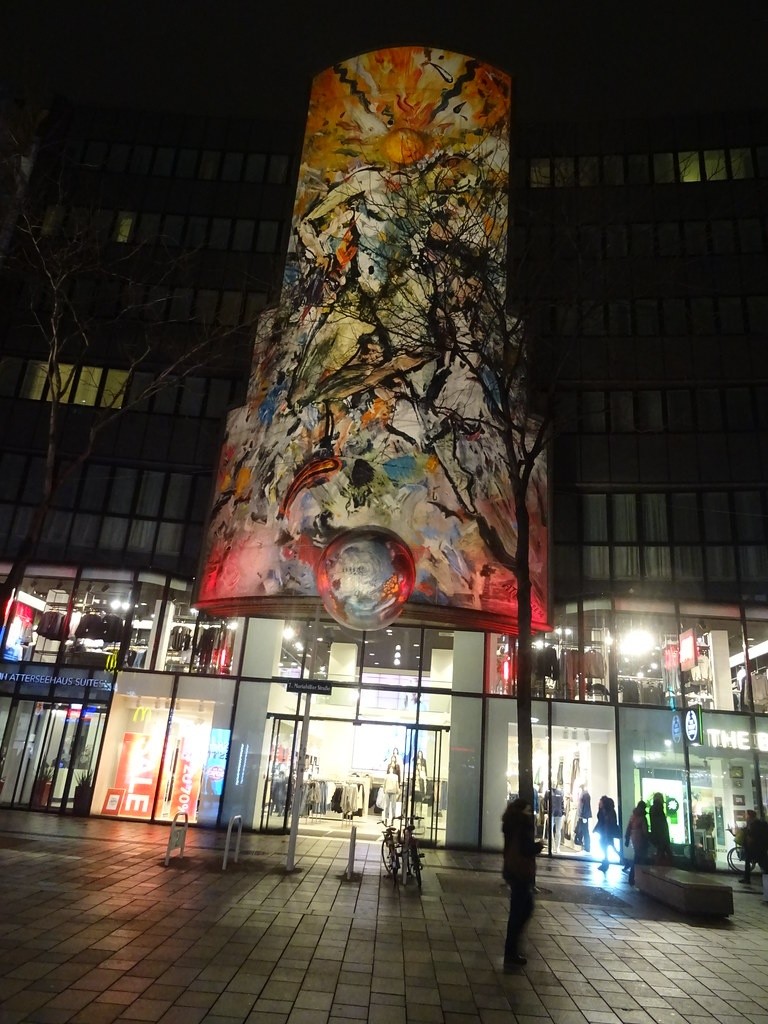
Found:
[504,953,527,966]
[739,878,751,884]
[760,900,768,904]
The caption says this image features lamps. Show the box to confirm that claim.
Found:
[56,583,63,590]
[31,582,38,587]
[698,620,706,630]
[101,584,110,592]
[86,584,95,592]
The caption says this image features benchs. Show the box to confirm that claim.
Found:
[634,863,734,918]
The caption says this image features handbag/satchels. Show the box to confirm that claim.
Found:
[647,841,658,860]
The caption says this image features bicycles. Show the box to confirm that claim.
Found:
[399,814,425,888]
[725,824,756,875]
[376,815,404,884]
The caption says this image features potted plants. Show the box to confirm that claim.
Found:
[31,769,54,806]
[72,771,94,811]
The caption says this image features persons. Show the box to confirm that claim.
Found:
[625,790,675,886]
[556,751,581,796]
[381,746,427,823]
[507,779,593,855]
[501,797,544,971]
[593,794,631,873]
[738,810,768,884]
